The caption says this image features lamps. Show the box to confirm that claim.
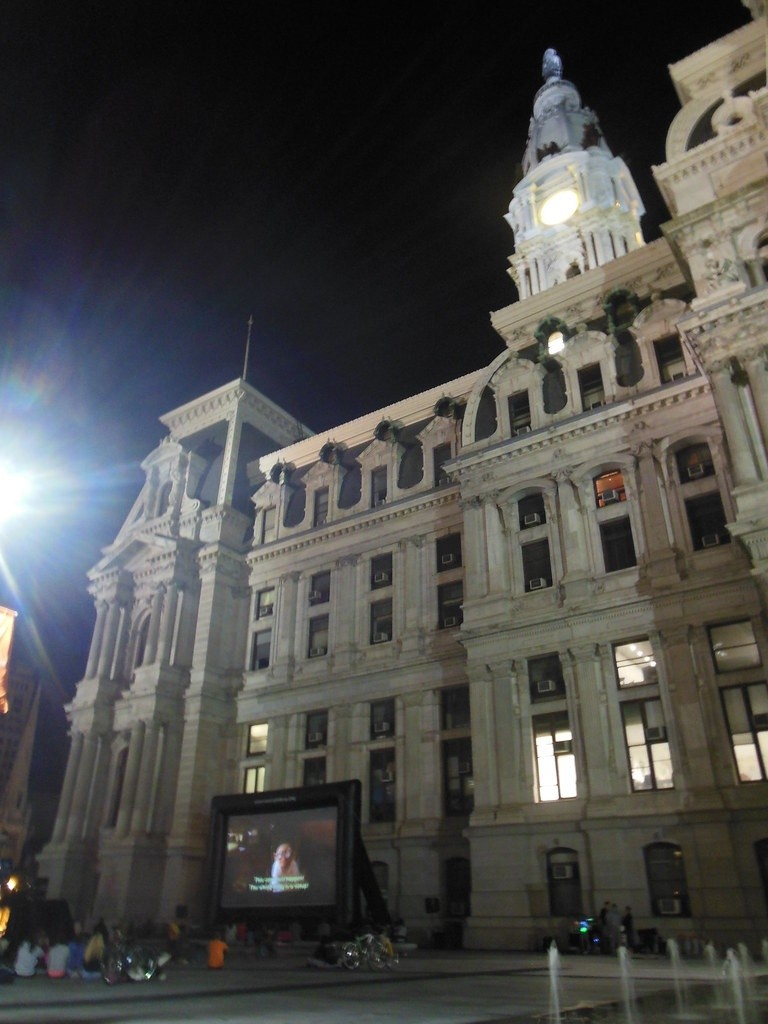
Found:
[517,426,531,436]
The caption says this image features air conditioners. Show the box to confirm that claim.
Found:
[537,680,556,692]
[444,617,458,627]
[442,553,456,564]
[439,478,450,487]
[375,573,388,582]
[589,399,604,410]
[380,772,393,782]
[525,513,541,525]
[375,722,389,732]
[457,763,469,773]
[309,732,322,741]
[375,499,385,508]
[645,726,667,740]
[553,740,572,753]
[259,607,271,617]
[552,865,572,878]
[671,372,686,382]
[307,590,321,601]
[529,578,546,590]
[702,533,719,547]
[310,648,324,656]
[753,714,768,728]
[659,899,682,915]
[602,490,619,503]
[316,520,326,527]
[687,464,704,478]
[373,633,388,642]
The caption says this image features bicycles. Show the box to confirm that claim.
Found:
[101,933,157,982]
[341,925,400,971]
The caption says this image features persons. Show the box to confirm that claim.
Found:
[599,901,632,956]
[0,919,408,986]
